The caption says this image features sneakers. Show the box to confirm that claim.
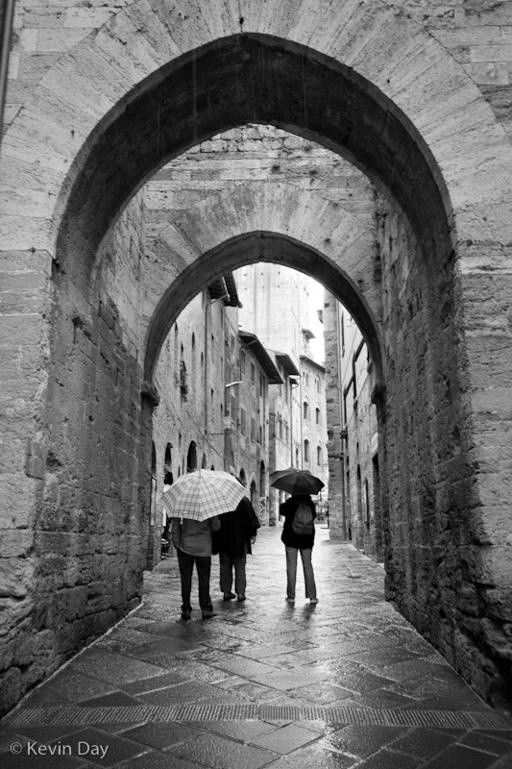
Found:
[309,596,319,604]
[175,613,193,624]
[284,596,295,604]
[236,592,247,602]
[222,590,235,601]
[201,610,217,620]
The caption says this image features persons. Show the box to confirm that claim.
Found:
[212,496,261,601]
[279,496,318,605]
[172,516,221,620]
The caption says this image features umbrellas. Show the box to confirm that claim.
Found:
[161,469,248,522]
[270,468,325,496]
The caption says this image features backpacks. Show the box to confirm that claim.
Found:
[290,502,314,536]
